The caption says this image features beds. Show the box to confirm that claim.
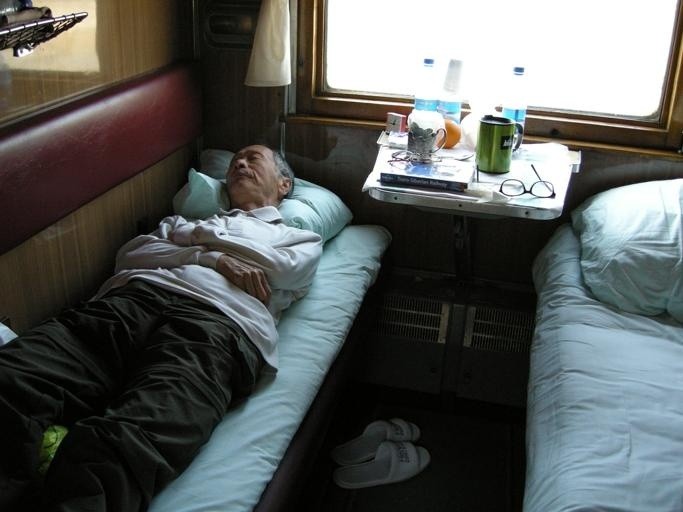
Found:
[2,174,396,512]
[522,156,683,512]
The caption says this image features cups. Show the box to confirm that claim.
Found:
[474,117,525,174]
[405,122,447,164]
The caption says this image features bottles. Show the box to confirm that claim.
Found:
[414,57,439,111]
[502,65,527,137]
[438,58,463,121]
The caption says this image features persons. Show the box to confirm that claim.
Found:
[0,145,326,512]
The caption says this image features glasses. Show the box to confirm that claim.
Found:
[500,164,556,198]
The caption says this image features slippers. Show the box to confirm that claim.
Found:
[333,440,431,489]
[330,418,420,466]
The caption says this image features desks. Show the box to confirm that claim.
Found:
[365,119,578,222]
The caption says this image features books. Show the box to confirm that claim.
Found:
[379,158,476,193]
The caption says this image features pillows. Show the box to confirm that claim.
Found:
[572,175,682,322]
[175,148,352,248]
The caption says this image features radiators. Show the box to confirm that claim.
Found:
[373,287,536,354]
